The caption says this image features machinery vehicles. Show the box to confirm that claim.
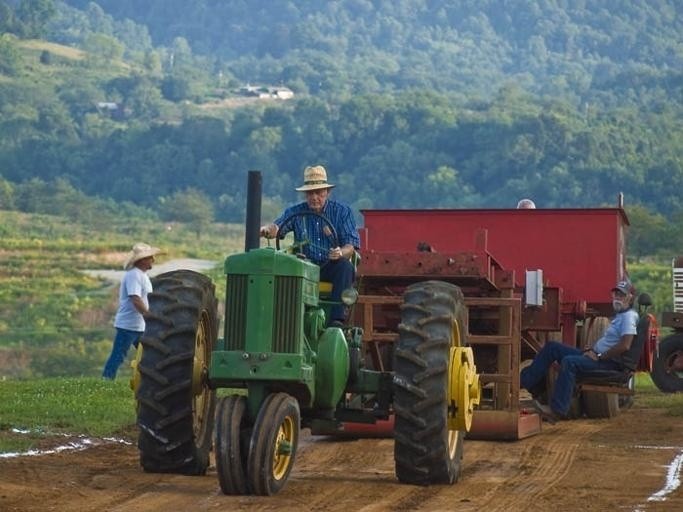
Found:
[136,190,634,496]
[648,256,682,391]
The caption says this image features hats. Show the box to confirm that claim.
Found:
[611,281,636,294]
[124,243,160,270]
[295,165,336,192]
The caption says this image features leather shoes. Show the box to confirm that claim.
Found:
[534,398,560,424]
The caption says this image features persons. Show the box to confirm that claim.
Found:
[516,198,536,209]
[258,166,360,327]
[519,281,640,426]
[100,242,168,379]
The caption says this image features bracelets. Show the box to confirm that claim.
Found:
[596,352,602,361]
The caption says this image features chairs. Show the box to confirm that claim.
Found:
[575,293,653,417]
[286,246,334,294]
